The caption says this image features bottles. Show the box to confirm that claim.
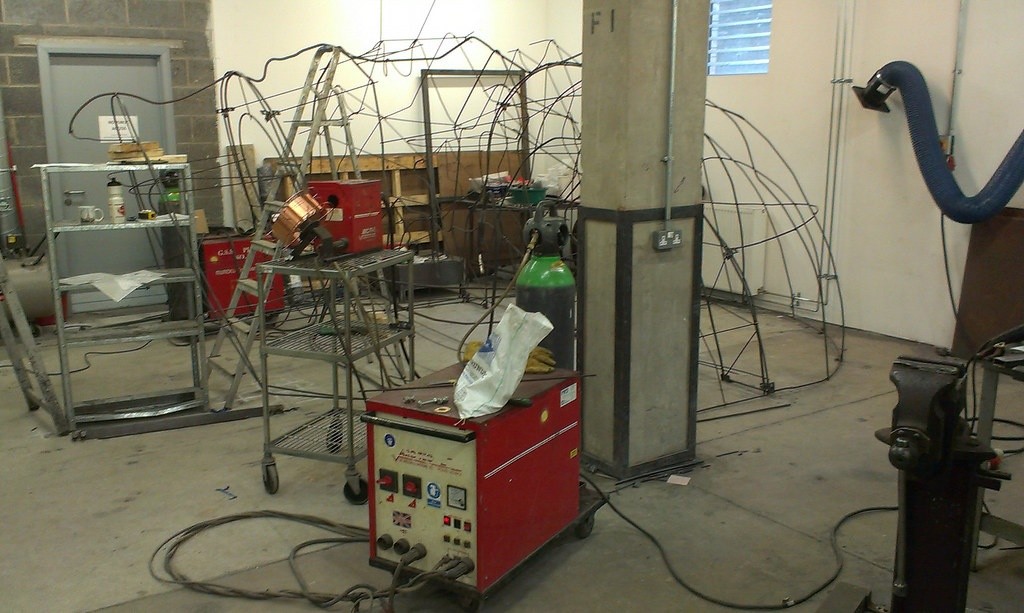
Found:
[107,178,126,225]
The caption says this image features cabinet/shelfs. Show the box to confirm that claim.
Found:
[256,249,415,506]
[30,157,209,443]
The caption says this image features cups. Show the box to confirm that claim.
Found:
[78,205,104,225]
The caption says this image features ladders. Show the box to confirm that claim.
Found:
[198,43,406,410]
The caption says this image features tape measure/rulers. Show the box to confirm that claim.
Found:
[138,208,157,219]
